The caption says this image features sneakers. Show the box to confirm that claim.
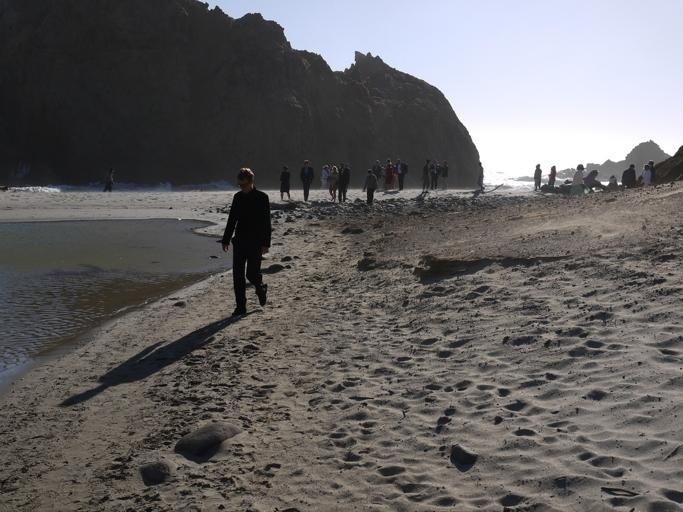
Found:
[232,307,247,316]
[256,285,266,306]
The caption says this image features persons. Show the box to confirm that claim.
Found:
[279,164,291,201]
[221,167,272,316]
[534,160,656,196]
[477,162,486,194]
[320,157,449,205]
[300,160,314,204]
[103,167,114,192]
